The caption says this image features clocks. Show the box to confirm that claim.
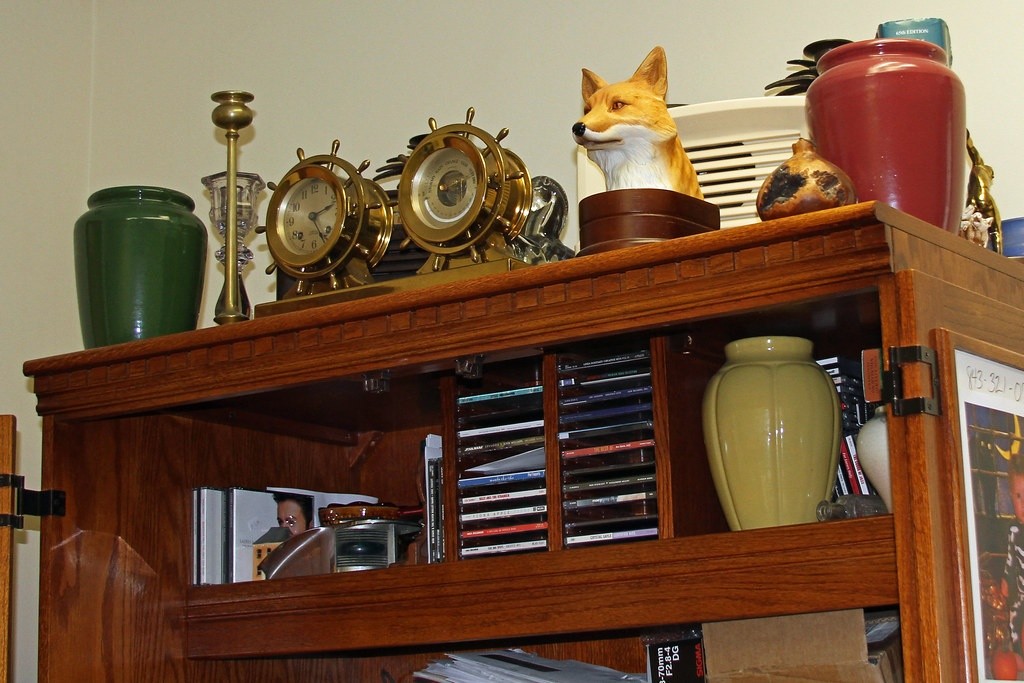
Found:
[250,138,396,298]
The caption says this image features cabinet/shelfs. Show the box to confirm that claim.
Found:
[25,70,1023,678]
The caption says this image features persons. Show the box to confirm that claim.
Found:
[1002,452,1024,671]
[272,493,316,536]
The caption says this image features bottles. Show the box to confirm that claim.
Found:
[816,494,888,522]
